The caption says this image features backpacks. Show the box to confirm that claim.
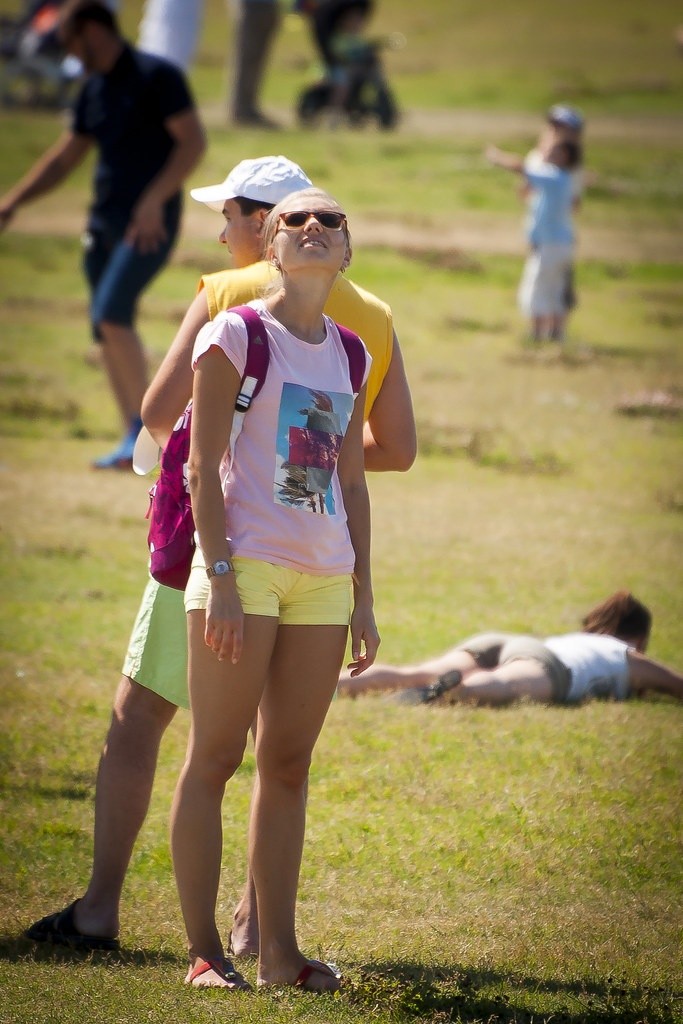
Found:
[142,307,365,593]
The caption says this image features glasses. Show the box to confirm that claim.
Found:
[271,211,349,242]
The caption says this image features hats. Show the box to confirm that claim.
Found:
[190,155,313,212]
[543,105,583,132]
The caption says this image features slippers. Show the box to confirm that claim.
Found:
[226,924,258,957]
[21,898,121,951]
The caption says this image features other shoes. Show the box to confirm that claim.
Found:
[389,668,463,708]
[231,108,278,129]
[90,421,141,472]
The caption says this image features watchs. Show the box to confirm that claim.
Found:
[206,562,236,578]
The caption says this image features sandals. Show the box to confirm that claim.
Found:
[254,961,349,990]
[182,952,254,991]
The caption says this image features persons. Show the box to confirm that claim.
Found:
[1,1,402,131]
[487,101,588,350]
[14,155,418,959]
[172,187,381,992]
[335,590,683,705]
[0,0,205,470]
